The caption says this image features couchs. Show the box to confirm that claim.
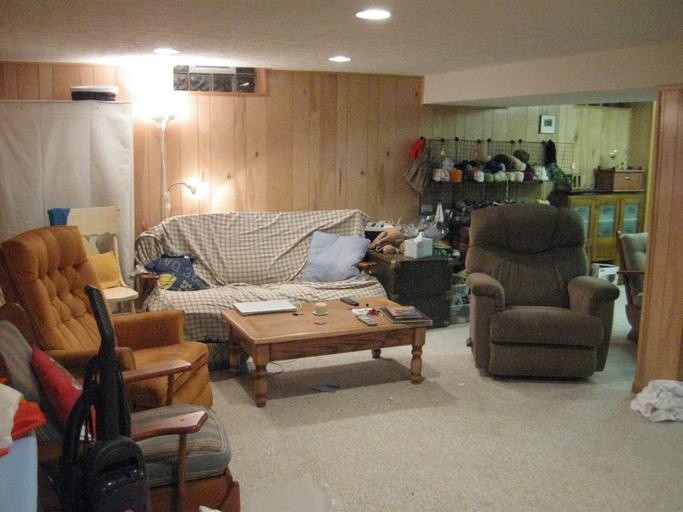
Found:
[138,209,388,371]
[0,225,213,409]
[465,206,620,382]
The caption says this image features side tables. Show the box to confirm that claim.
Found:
[368,246,453,329]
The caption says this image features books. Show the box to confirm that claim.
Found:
[379,305,431,324]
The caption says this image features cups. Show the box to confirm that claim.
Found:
[311,302,329,325]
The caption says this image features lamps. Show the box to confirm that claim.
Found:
[152,109,206,218]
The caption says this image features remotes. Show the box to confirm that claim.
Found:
[340,297,359,307]
[355,315,376,328]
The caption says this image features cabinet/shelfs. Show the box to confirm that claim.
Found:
[419,141,574,252]
[594,195,618,265]
[568,195,594,262]
[0,97,135,286]
[172,66,269,97]
[620,194,645,232]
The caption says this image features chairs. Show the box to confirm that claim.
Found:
[0,302,242,512]
[51,206,138,315]
[616,230,649,345]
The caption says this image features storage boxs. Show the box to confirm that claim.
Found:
[593,165,646,193]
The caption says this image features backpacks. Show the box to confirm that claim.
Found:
[47,357,152,512]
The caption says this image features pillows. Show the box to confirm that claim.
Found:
[144,255,211,291]
[302,231,370,283]
